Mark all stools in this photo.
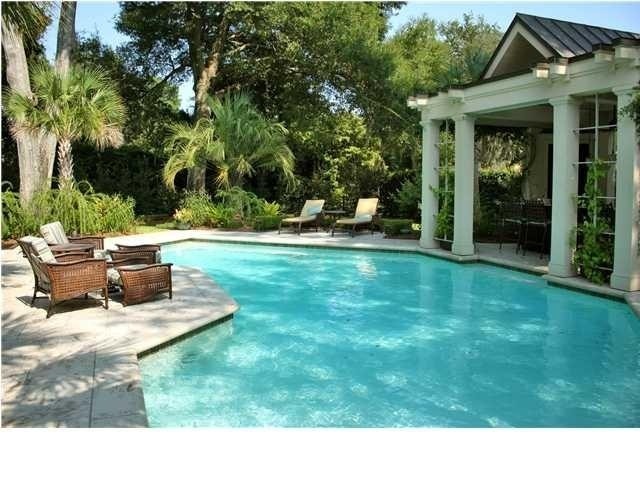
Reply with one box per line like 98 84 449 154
106 243 174 307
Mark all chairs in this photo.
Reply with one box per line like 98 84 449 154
499 198 551 259
278 197 380 238
18 220 108 319
